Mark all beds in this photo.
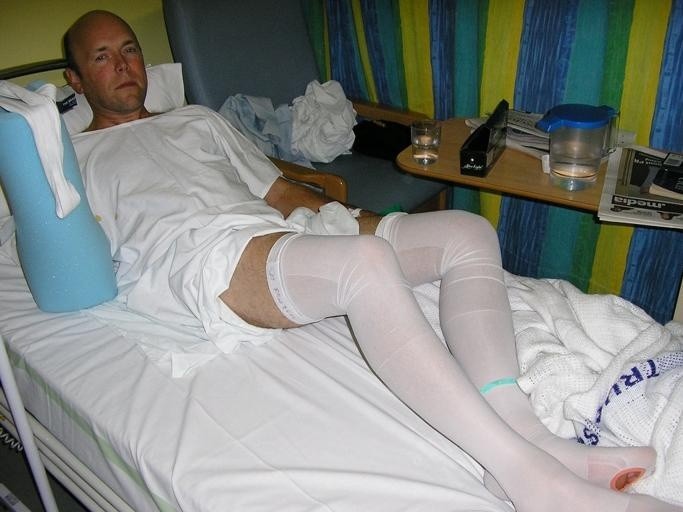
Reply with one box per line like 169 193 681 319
0 59 683 512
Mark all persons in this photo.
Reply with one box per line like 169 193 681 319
611 205 632 212
657 212 680 220
61 8 683 512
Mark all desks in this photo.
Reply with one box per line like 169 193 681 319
397 117 606 210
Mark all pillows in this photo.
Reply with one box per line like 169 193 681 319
55 63 185 133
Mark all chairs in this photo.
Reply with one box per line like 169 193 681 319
163 0 451 217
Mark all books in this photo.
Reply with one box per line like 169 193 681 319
648 152 683 201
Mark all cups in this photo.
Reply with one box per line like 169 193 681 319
410 117 441 166
532 101 619 194
